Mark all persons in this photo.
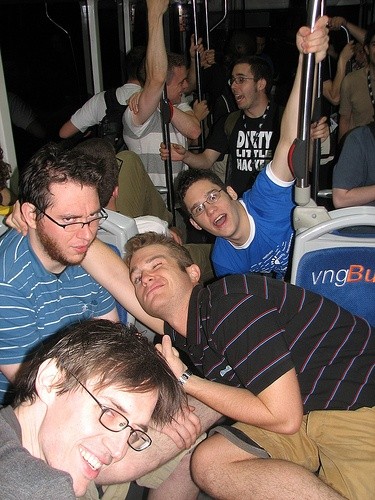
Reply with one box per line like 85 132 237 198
0 142 179 410
4 199 375 500
0 0 375 282
0 319 223 500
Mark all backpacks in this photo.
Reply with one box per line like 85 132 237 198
94 89 128 153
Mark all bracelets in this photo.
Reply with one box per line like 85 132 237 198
175 369 192 386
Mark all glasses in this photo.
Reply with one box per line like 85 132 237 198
189 187 225 218
61 364 152 453
38 208 109 233
227 76 254 86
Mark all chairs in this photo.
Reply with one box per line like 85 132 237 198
290 214 375 327
